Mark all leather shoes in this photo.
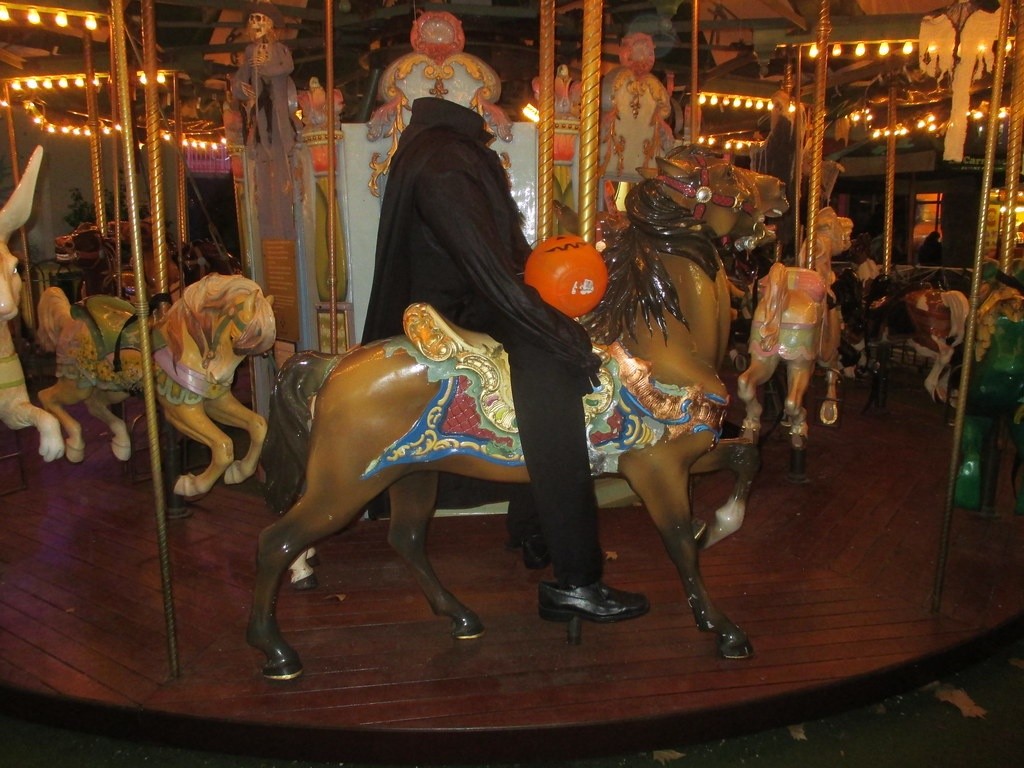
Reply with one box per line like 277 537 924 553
537 581 651 623
523 536 550 570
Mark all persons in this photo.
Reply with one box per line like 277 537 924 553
361 94 650 623
918 230 943 268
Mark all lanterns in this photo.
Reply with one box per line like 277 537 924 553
523 233 609 318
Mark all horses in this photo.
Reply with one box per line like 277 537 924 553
238 146 791 682
724 206 1024 521
33 271 278 497
53 218 235 307
